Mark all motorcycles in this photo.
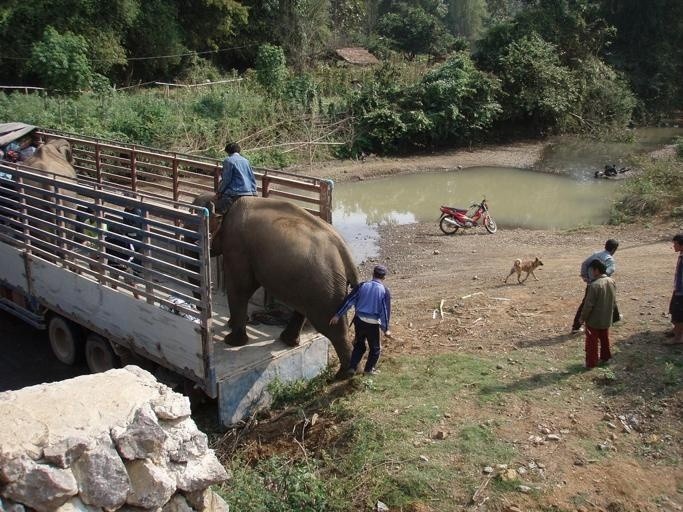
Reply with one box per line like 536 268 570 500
438 194 497 235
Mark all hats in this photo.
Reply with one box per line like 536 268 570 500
374 265 386 274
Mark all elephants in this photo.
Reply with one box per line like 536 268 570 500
8 138 83 276
183 190 365 380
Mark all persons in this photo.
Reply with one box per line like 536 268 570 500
194 142 258 255
577 259 617 369
662 233 682 346
328 264 390 376
80 205 107 288
118 192 143 283
106 224 141 301
570 238 623 334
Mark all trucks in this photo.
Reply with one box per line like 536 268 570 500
1 120 349 435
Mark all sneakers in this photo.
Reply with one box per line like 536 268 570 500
571 328 583 333
347 369 356 376
664 327 678 335
364 368 380 375
664 336 682 345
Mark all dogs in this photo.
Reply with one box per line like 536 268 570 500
504 256 544 284
